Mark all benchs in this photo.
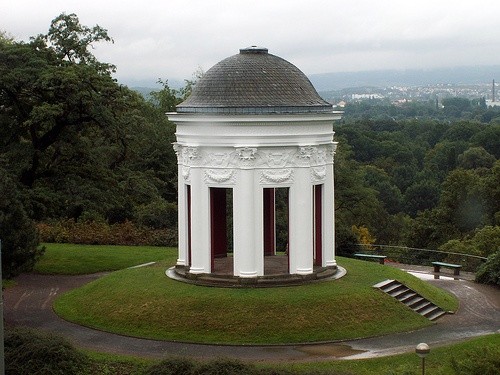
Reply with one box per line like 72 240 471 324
432 260 462 275
354 253 386 264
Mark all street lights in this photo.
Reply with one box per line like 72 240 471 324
416 343 430 375
